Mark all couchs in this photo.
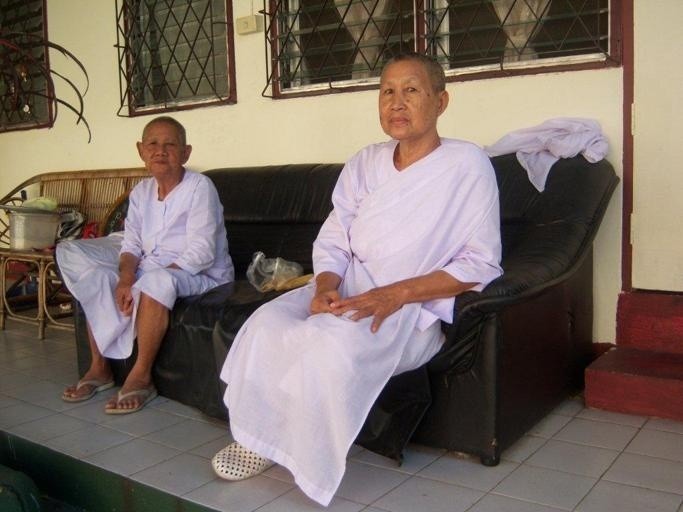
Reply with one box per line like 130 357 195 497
52 147 619 465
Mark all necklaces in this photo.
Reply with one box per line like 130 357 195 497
157 178 181 200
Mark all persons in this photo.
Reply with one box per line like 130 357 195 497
210 50 505 507
53 116 236 414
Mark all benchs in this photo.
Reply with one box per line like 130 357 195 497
0 165 159 342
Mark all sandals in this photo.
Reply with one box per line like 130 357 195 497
212 441 276 482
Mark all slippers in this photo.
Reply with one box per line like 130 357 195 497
104 386 157 414
62 376 114 402
